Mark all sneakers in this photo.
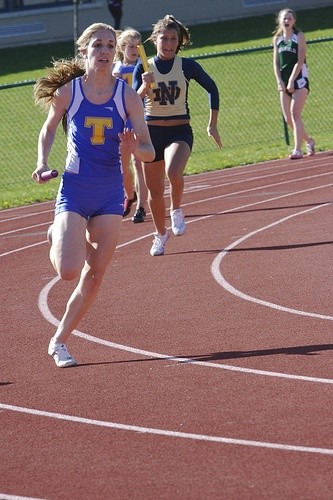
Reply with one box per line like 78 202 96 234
149 226 169 257
123 191 137 217
47 338 77 368
131 206 146 223
289 149 303 159
170 208 186 236
306 138 315 156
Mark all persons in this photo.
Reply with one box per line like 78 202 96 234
111 28 148 223
107 0 122 28
272 9 315 160
30 23 155 368
132 15 222 256
